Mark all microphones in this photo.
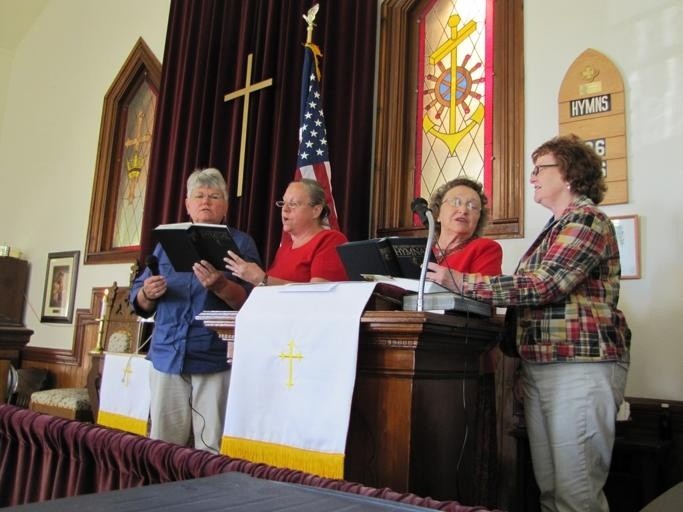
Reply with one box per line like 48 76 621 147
147 255 160 278
411 196 431 230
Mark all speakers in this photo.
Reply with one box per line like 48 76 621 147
604 395 683 511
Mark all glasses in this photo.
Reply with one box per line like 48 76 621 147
274 200 320 209
440 196 481 214
530 164 558 177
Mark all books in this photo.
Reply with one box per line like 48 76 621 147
151 221 245 274
399 290 494 321
335 235 438 282
358 272 455 303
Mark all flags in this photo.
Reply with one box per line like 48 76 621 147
277 40 342 249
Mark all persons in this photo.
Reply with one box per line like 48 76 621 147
127 166 264 458
221 177 350 288
419 173 503 279
419 132 633 512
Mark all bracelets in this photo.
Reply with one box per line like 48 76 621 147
258 272 268 287
141 288 155 302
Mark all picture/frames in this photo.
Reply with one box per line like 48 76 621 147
608 214 641 280
40 251 80 324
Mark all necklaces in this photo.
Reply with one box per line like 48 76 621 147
433 234 476 259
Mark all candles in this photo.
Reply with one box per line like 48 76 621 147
100 289 109 320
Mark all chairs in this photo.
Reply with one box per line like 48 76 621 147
29 330 130 423
16 369 49 409
